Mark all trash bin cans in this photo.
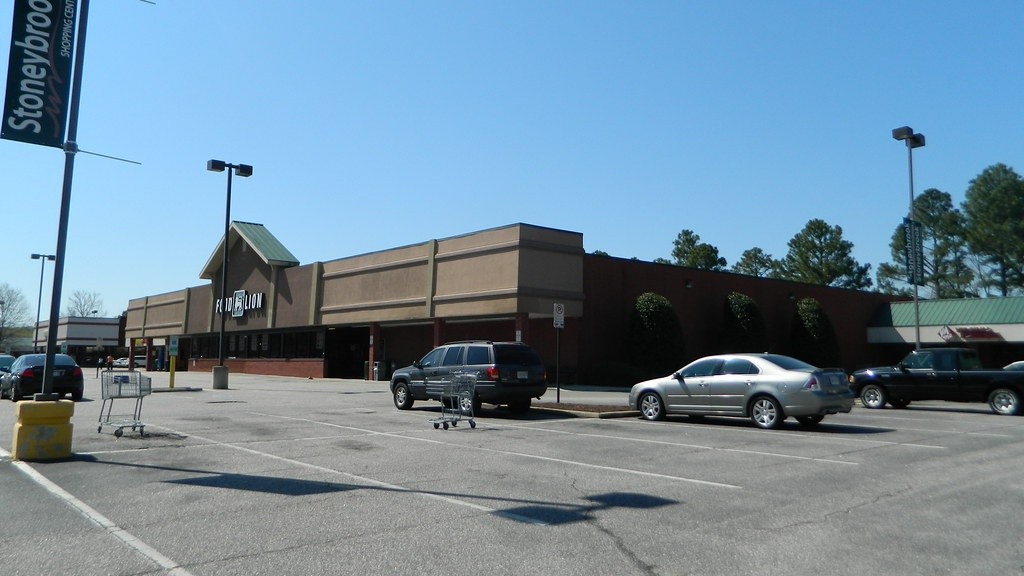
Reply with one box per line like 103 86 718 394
391 362 398 375
364 361 369 380
164 360 170 372
374 361 385 381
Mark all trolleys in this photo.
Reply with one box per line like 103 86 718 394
425 370 477 431
95 370 153 438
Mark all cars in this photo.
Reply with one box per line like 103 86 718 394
0 354 16 378
628 352 854 431
1003 361 1024 371
0 354 85 401
113 357 127 367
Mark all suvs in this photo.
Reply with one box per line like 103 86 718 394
392 340 550 412
851 347 1024 418
127 356 147 368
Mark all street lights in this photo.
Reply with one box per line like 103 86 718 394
890 125 926 350
30 254 57 352
208 157 254 391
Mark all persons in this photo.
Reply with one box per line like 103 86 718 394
107 353 113 370
98 353 105 369
